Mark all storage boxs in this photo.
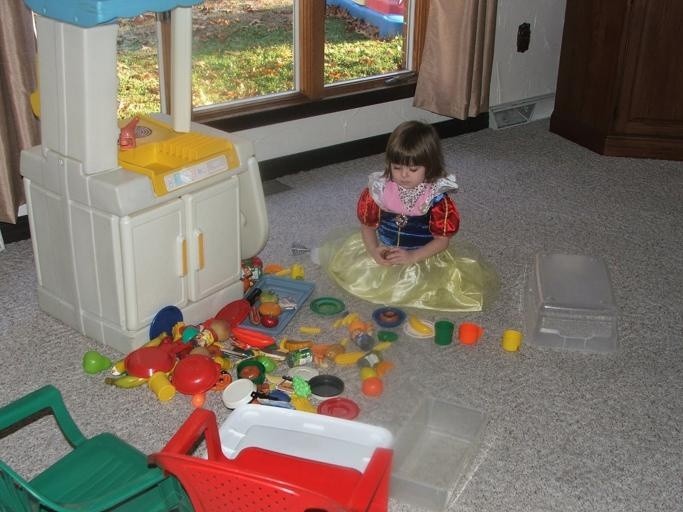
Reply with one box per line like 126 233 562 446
522 252 621 354
384 395 488 512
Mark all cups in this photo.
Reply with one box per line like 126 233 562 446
459 324 478 344
503 330 523 352
435 321 453 345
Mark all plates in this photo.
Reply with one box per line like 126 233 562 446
403 319 436 338
371 307 404 327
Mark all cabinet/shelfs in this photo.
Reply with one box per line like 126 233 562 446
21 116 244 353
548 1 682 162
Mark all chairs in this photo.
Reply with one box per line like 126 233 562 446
149 403 392 511
0 385 194 511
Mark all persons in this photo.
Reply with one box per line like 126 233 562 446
319 119 498 312
